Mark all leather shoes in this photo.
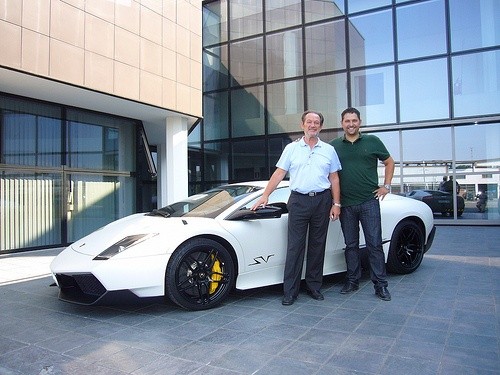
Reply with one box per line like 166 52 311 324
282 294 297 305
308 290 324 299
340 281 359 294
375 287 391 301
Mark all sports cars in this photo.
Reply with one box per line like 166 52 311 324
49 180 437 311
401 189 466 218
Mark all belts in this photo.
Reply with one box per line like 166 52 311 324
291 189 329 197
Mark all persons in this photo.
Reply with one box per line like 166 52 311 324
250 111 343 305
476 190 488 213
294 107 395 302
438 175 462 217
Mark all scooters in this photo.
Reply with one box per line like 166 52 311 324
474 194 487 213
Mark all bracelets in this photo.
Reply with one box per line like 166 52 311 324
383 184 391 189
333 202 341 206
